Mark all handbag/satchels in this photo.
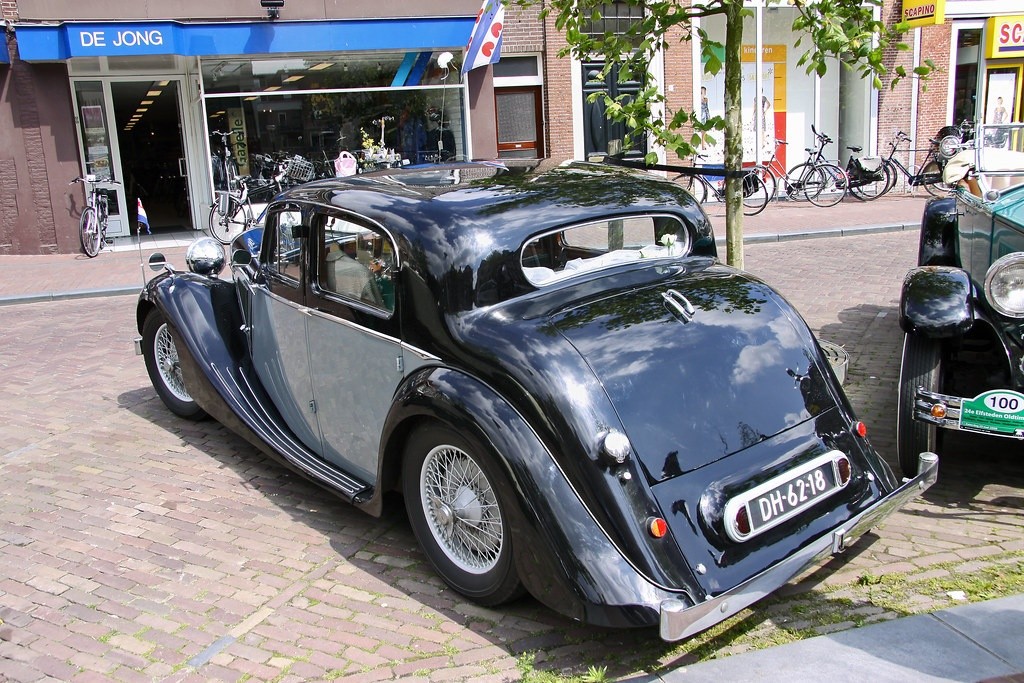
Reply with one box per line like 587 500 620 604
335 150 357 177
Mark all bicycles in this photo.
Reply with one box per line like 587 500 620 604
667 149 768 213
750 139 848 206
802 121 1012 202
67 175 122 258
200 128 352 248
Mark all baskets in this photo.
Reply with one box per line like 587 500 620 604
287 158 314 182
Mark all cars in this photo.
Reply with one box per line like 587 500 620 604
133 153 941 650
899 121 1024 485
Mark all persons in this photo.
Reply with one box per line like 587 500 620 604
991 97 1008 148
701 86 710 150
753 87 771 149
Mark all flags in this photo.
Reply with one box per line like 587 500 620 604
138 198 151 235
